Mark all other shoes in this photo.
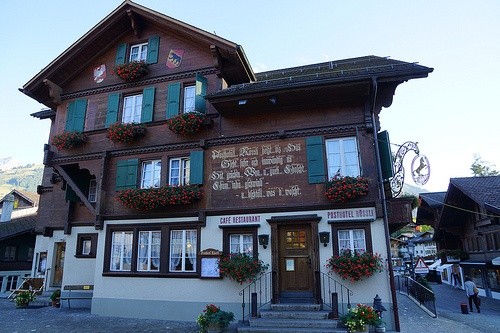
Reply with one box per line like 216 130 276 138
470 310 473 312
478 310 480 313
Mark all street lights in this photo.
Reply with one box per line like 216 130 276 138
406 240 416 281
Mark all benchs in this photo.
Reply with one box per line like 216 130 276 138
56 285 94 310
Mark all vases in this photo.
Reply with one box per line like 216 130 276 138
207 324 222 333
356 325 369 333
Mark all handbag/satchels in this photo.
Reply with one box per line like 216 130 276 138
473 287 479 294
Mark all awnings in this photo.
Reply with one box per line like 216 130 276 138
436 264 453 271
428 259 442 270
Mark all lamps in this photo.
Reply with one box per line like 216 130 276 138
319 232 330 247
259 235 269 249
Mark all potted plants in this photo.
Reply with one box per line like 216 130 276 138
14 290 37 309
50 290 61 308
376 318 385 333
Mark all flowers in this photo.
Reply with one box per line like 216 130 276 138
114 184 201 211
323 248 383 282
338 302 379 333
322 172 369 203
105 121 147 145
169 110 207 138
51 131 89 152
217 253 270 285
196 303 236 333
110 59 149 81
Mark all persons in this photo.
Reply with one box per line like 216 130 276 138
464 276 481 313
